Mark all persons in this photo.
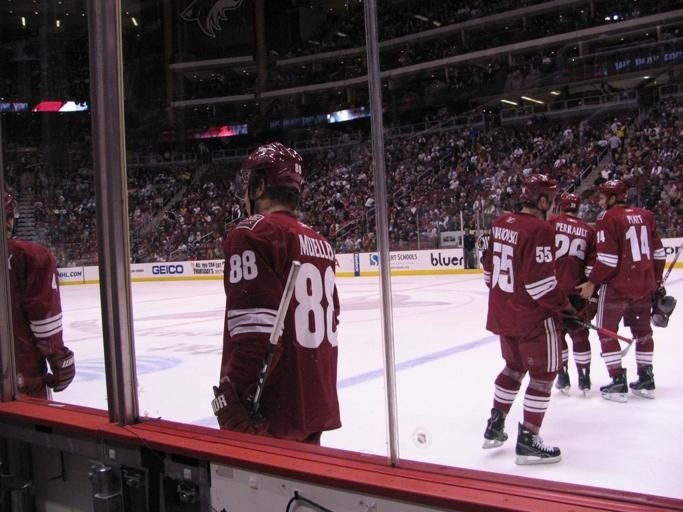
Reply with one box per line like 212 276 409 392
544 190 597 398
608 132 622 159
0 190 76 401
573 178 665 404
210 139 342 446
458 224 477 269
481 174 579 467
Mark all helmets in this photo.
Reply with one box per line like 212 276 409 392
519 173 558 210
0 187 16 234
559 190 581 212
236 140 307 204
597 178 628 203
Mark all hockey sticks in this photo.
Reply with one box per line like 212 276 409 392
248 259 301 419
564 318 633 358
621 248 682 357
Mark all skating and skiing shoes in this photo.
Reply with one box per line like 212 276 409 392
515 426 563 464
599 368 629 403
628 364 656 400
483 409 510 450
577 368 593 399
554 364 572 394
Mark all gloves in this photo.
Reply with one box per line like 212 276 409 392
562 311 579 330
209 375 255 434
42 346 76 393
